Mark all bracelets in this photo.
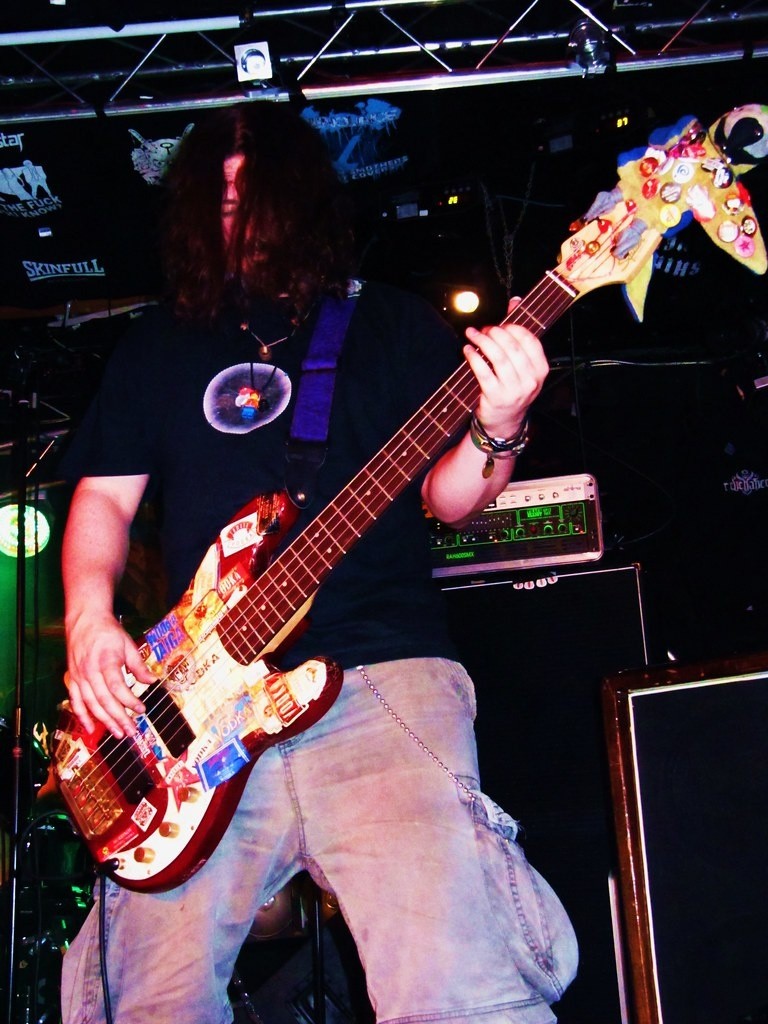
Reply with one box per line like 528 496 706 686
470 416 528 479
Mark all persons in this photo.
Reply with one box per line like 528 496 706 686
47 100 579 1024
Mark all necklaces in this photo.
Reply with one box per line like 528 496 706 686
241 308 312 418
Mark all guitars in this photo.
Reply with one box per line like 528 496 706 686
36 103 768 894
